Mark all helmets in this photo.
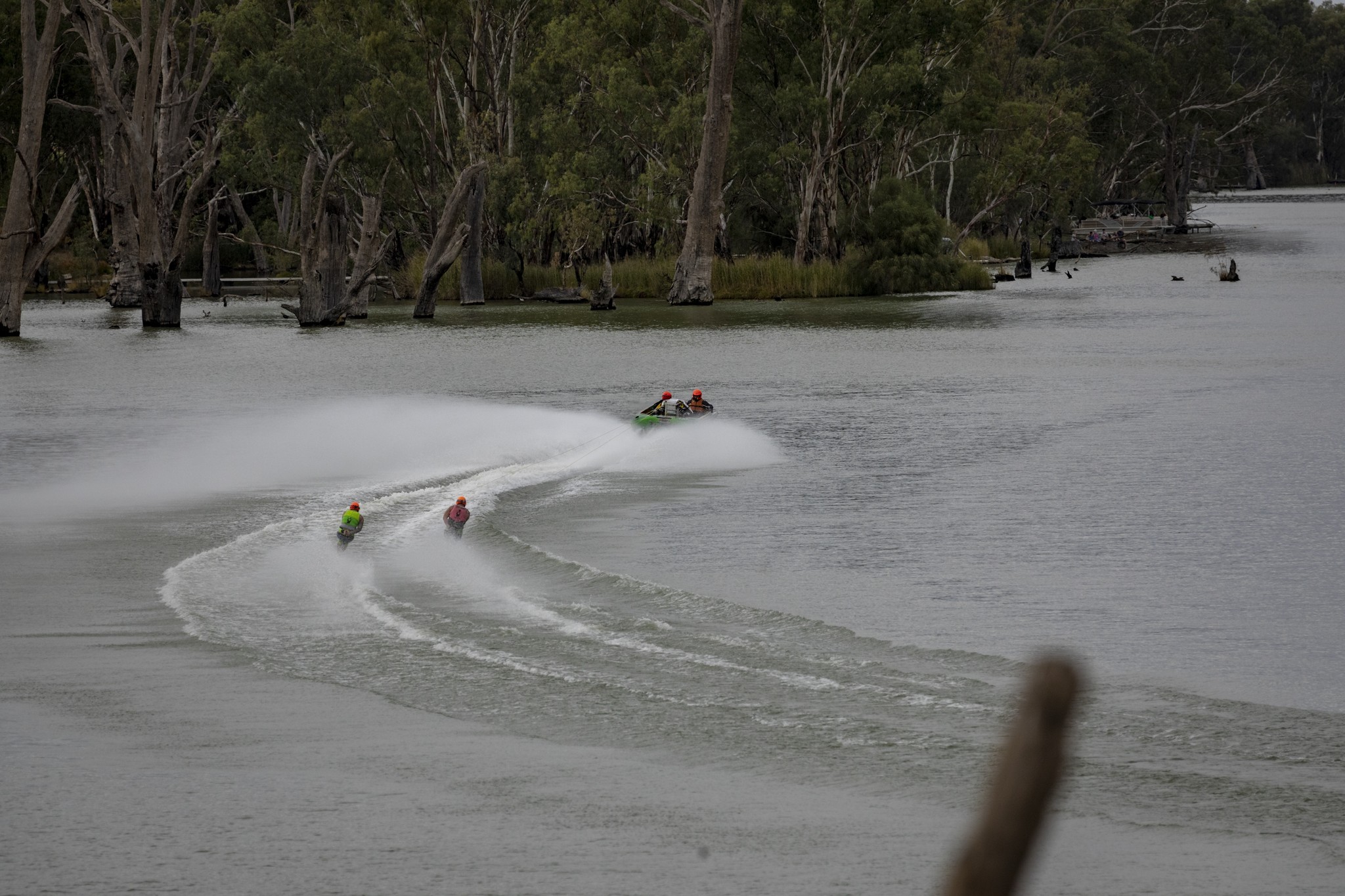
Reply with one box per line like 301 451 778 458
350 502 360 512
662 392 672 400
456 497 466 507
692 389 702 401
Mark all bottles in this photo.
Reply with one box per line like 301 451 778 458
1137 234 1138 239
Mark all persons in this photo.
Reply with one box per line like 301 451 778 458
443 496 470 541
653 392 672 416
683 389 714 418
1135 212 1140 217
1071 229 1079 241
337 502 364 552
1149 213 1154 219
1160 211 1166 219
1087 227 1127 249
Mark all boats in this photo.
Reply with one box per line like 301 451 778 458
630 399 719 430
